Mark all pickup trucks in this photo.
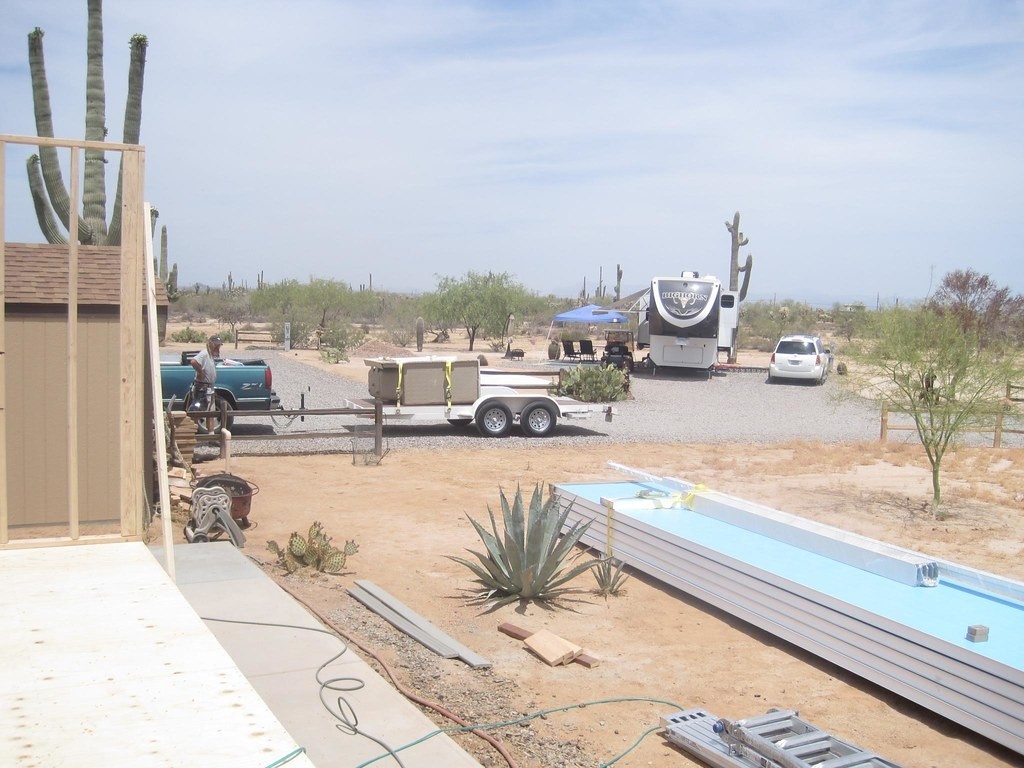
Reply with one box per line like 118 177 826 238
159 358 281 433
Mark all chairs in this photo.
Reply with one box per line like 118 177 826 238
562 340 581 363
579 340 597 363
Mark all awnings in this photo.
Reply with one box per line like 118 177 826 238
592 287 650 312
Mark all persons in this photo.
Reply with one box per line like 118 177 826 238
925 368 936 391
191 335 223 447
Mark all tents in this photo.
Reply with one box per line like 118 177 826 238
540 304 632 360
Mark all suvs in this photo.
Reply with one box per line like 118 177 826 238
765 334 831 387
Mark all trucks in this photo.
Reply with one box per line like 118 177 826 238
639 271 739 379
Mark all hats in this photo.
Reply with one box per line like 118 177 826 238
210 335 224 346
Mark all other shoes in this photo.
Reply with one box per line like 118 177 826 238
209 441 220 446
